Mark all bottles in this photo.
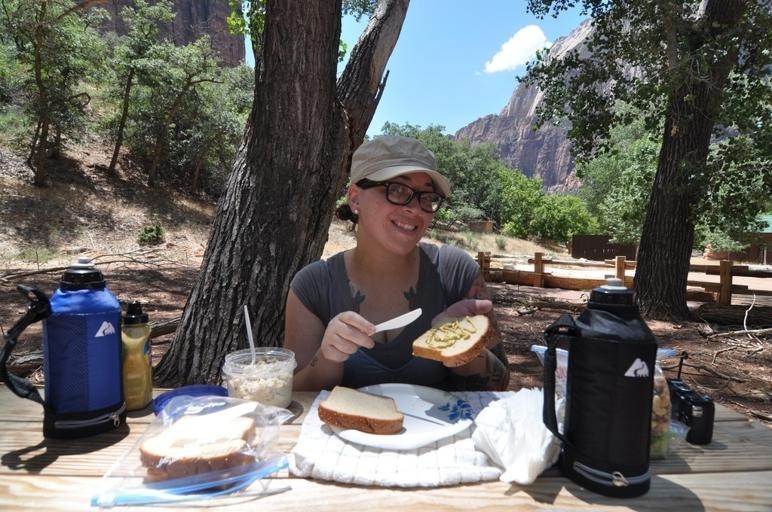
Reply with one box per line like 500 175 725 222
41 256 125 438
121 298 155 410
561 276 661 497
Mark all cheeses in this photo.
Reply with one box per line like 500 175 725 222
228 358 294 409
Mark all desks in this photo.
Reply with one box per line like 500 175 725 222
3 377 770 510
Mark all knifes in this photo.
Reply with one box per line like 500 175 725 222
365 306 424 337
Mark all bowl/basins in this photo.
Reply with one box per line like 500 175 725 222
224 347 305 412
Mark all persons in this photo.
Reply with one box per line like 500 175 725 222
282 133 511 392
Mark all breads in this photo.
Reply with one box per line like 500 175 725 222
317 384 404 434
412 314 492 368
138 399 263 492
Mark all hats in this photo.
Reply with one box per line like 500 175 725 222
349 136 450 200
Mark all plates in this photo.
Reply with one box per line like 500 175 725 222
332 383 473 451
153 384 230 416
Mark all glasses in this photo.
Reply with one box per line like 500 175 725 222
360 181 445 213
